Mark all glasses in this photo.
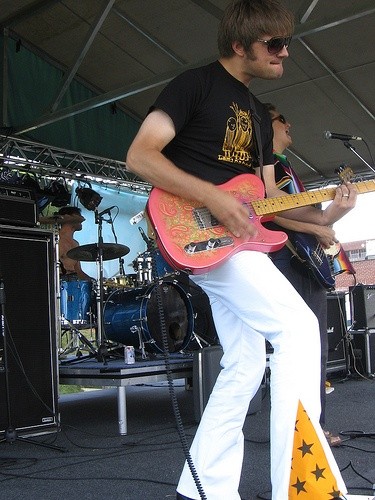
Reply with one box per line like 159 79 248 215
256 35 292 55
272 114 285 124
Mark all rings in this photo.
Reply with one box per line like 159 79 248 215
343 194 349 197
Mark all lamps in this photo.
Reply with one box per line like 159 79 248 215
0 159 72 207
75 179 103 211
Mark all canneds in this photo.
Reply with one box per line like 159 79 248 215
124 346 136 364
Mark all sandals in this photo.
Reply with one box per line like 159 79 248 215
323 429 342 446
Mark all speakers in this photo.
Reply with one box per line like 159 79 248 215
349 285 375 329
348 330 375 377
0 225 60 439
326 292 350 376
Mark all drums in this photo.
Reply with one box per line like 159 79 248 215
57 276 102 332
41 212 86 226
133 245 182 286
102 273 135 293
106 281 198 356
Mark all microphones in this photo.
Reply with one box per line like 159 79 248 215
97 206 115 219
321 130 362 140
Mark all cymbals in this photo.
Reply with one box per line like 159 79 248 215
66 242 131 262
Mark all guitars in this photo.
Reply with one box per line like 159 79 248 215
143 173 375 275
285 164 357 290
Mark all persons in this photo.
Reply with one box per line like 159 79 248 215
54 207 92 279
125 0 357 500
257 101 339 448
